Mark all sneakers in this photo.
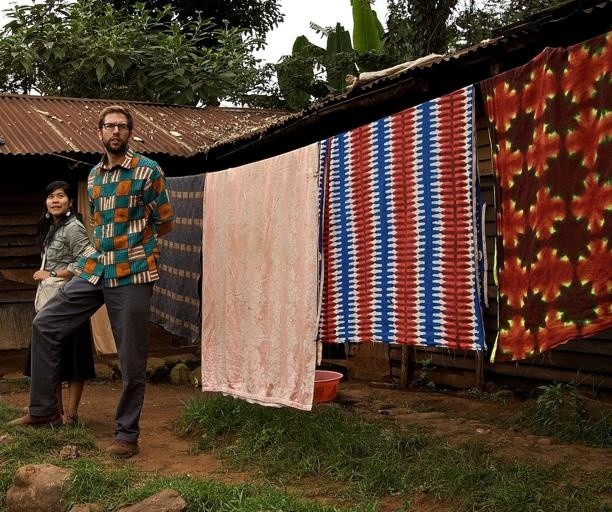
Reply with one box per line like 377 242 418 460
59 413 79 431
107 440 139 458
7 411 64 427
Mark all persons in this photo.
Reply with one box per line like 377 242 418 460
21 181 97 429
6 105 175 459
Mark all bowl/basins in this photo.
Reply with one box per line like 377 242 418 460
300 368 344 403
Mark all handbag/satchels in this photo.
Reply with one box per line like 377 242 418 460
34 276 68 313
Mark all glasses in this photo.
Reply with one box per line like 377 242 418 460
103 122 128 132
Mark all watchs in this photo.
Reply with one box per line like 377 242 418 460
50 270 57 278
